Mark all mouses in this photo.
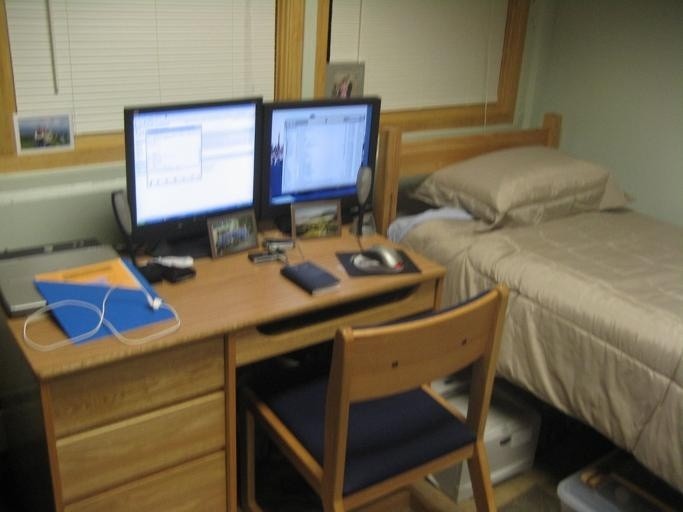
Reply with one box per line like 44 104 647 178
360 240 406 274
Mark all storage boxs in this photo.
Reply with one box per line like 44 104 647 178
427 383 543 503
557 453 681 512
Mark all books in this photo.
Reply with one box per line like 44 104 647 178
280 260 342 298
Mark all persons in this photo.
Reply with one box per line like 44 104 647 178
33 126 53 147
338 72 353 98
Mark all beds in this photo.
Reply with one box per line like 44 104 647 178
375 111 683 493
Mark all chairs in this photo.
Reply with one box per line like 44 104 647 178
239 283 509 512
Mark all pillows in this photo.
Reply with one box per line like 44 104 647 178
411 146 638 233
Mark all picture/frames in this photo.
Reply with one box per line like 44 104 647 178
13 110 75 157
206 208 259 259
324 63 365 100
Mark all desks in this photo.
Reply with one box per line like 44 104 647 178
0 224 447 511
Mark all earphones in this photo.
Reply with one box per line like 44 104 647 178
147 292 163 310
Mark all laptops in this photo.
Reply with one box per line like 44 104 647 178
1 242 122 320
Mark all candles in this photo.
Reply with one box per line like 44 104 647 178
290 199 342 240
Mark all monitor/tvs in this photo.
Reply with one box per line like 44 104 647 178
263 95 382 234
123 98 263 259
